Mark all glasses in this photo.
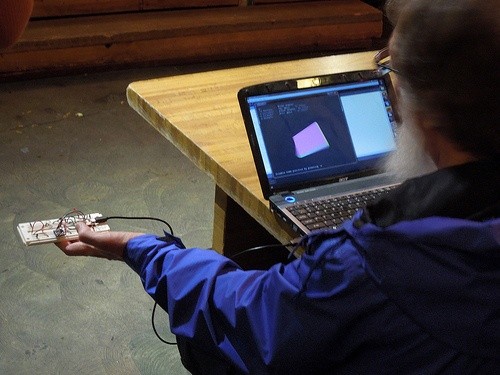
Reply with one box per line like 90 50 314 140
372 44 413 81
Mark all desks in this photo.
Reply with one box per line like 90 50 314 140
126 51 396 260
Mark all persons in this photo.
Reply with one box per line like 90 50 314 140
52 0 500 375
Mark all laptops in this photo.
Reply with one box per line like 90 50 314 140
237 69 405 237
